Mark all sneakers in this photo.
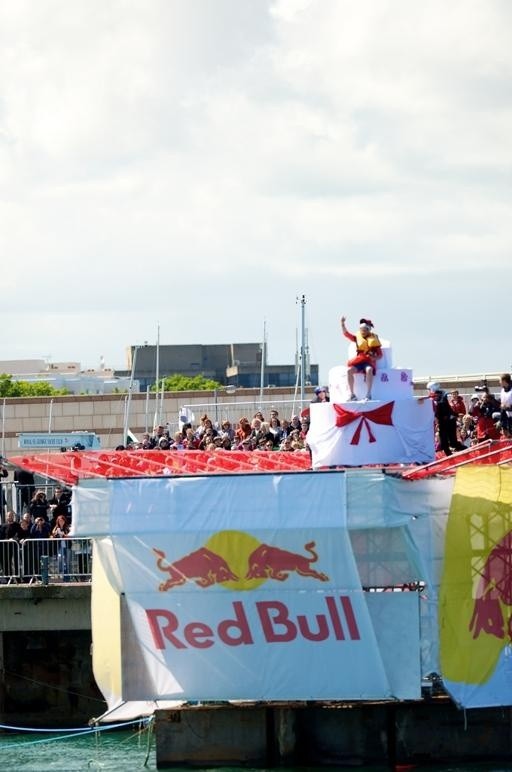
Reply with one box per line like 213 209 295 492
346 393 372 401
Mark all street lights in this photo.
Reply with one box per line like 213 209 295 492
215 385 236 422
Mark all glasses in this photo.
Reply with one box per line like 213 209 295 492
160 414 308 447
319 390 325 392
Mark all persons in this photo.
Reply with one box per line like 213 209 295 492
1 463 91 583
425 373 512 457
340 316 383 402
115 385 330 452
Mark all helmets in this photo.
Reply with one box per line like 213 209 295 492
426 381 440 393
314 386 326 393
359 322 372 331
